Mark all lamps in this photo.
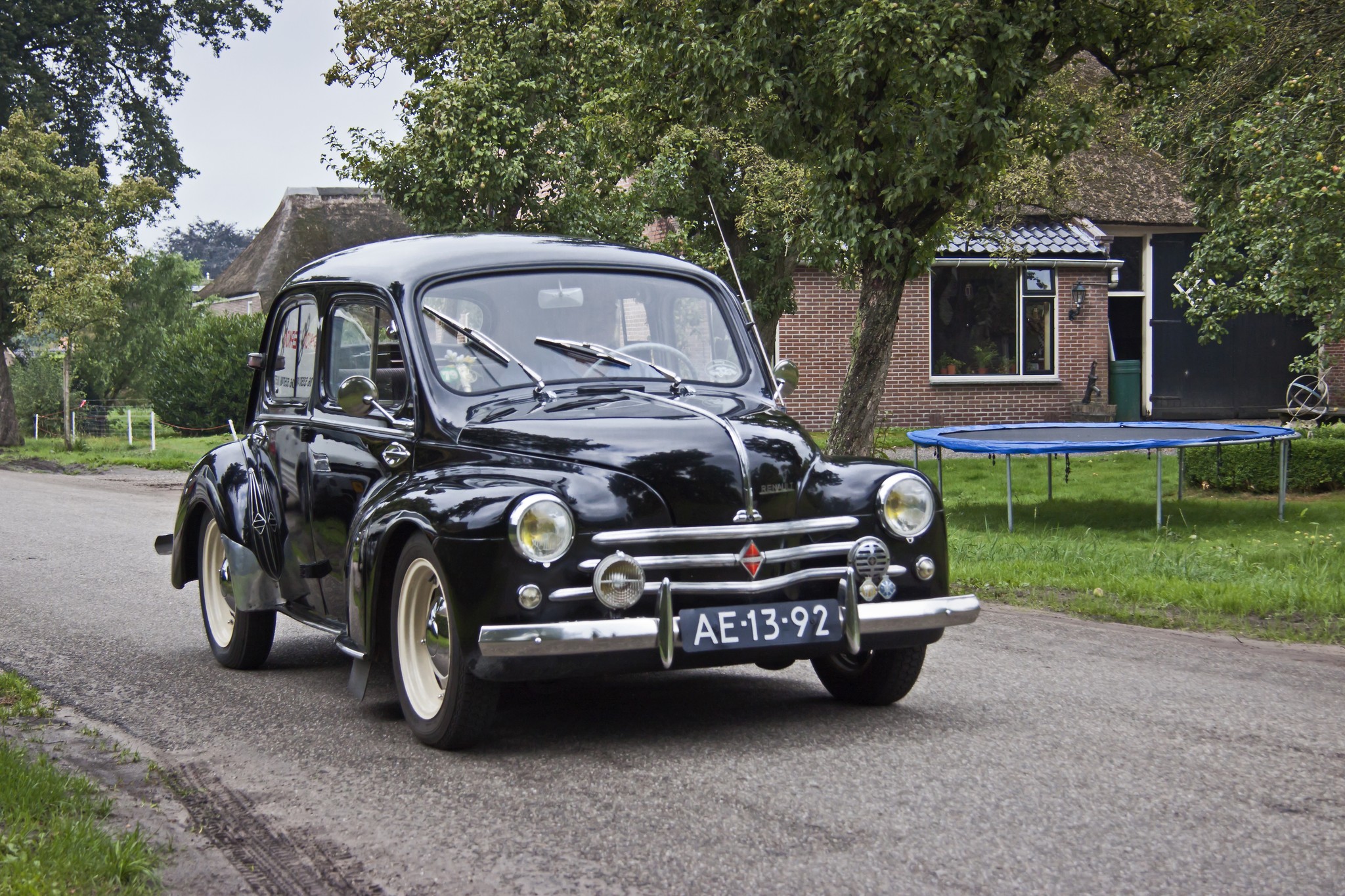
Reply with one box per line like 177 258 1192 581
1069 277 1087 321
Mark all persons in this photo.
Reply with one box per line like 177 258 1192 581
541 277 673 379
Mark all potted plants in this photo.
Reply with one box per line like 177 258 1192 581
930 340 1036 376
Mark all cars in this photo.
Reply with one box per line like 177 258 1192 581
155 195 979 752
